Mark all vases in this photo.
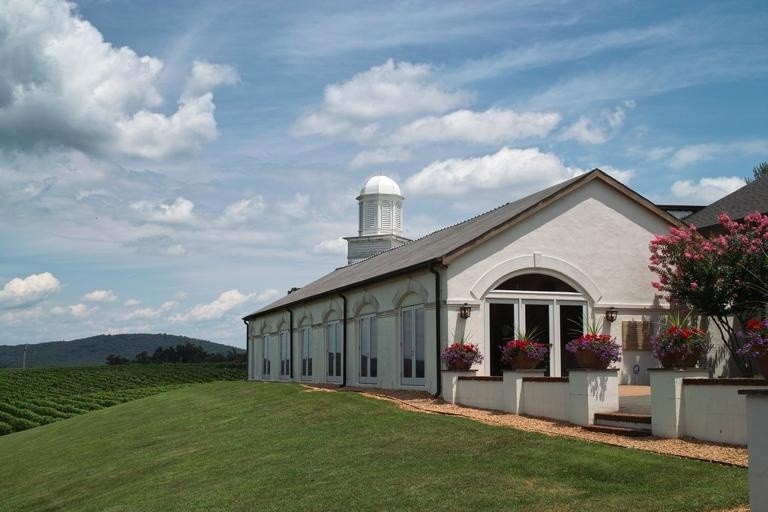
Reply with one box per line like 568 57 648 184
443 359 475 373
571 344 615 372
664 344 703 371
752 346 768 382
506 349 540 371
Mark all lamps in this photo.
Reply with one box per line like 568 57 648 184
459 301 473 320
604 305 619 323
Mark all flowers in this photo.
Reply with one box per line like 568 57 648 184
439 338 486 364
653 319 709 359
563 331 621 361
498 333 550 364
736 311 768 359
